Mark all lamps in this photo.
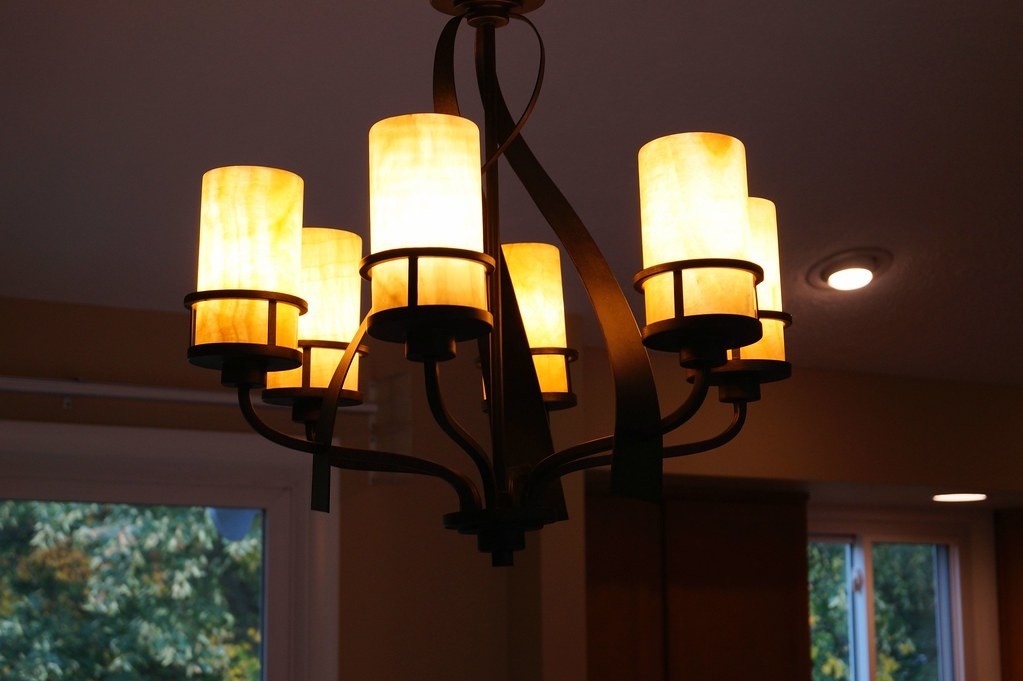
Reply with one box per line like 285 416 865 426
804 243 906 292
184 0 791 570
